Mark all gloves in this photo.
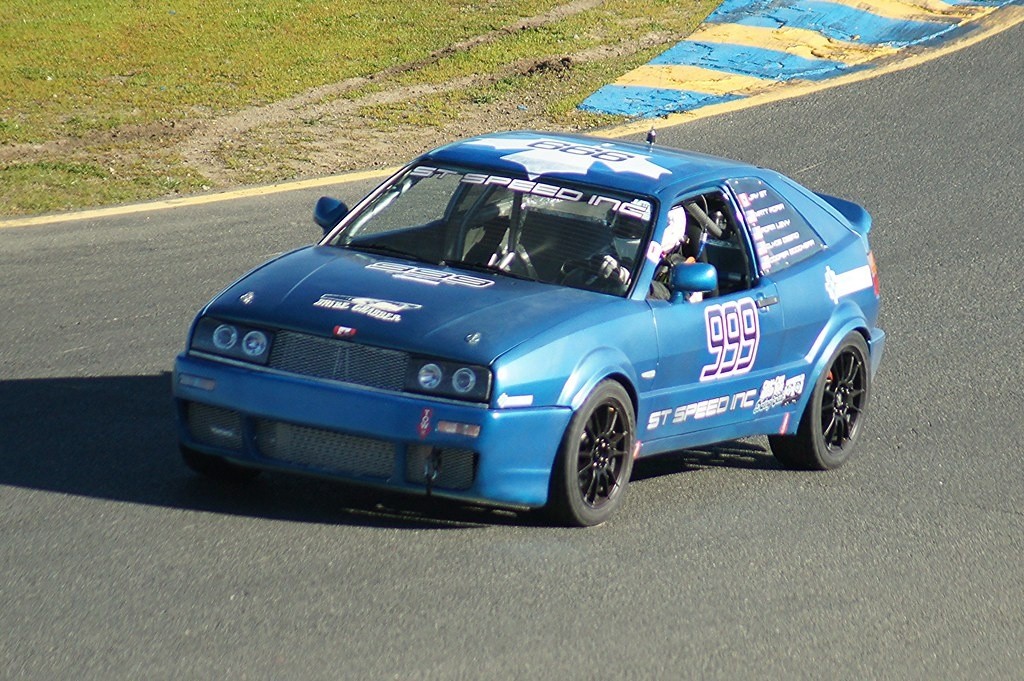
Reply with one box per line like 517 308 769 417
589 254 632 287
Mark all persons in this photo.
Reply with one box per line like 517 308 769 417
581 199 697 306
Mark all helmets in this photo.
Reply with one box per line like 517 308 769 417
606 199 687 265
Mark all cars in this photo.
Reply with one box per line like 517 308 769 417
169 127 888 529
